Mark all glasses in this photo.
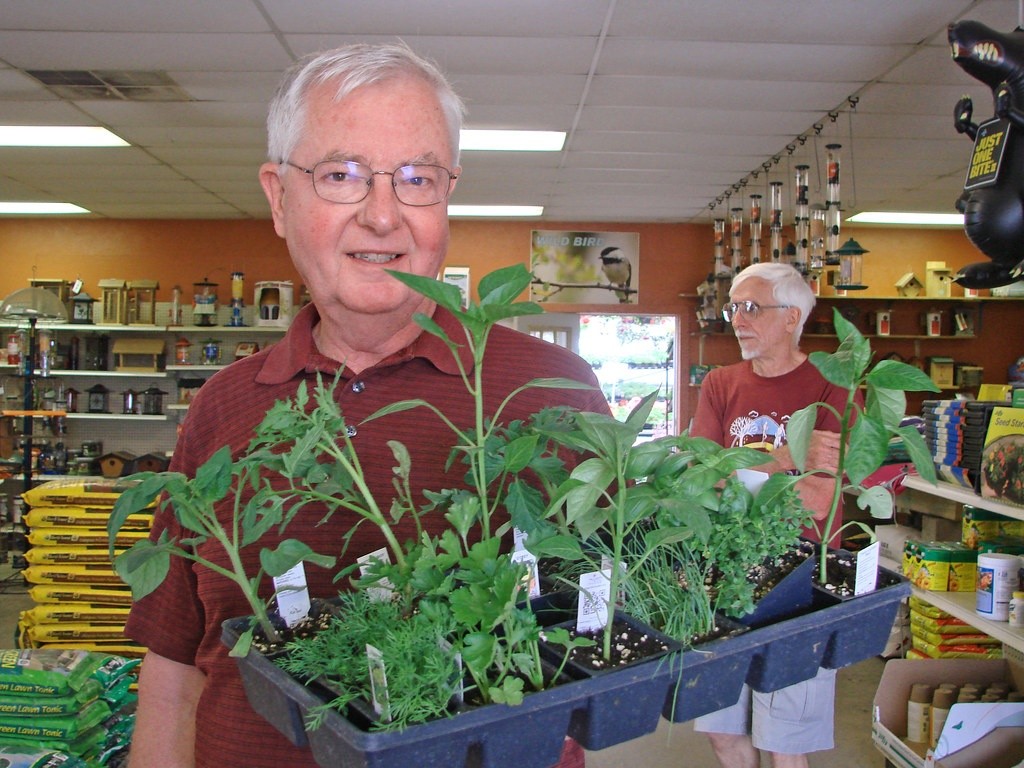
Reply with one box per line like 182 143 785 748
278 155 458 208
721 300 791 323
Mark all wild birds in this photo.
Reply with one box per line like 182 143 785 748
598 246 632 302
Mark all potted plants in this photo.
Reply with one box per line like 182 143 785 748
108 265 945 768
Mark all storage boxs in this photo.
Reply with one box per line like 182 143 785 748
872 657 1024 768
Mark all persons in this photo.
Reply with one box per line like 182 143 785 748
125 41 635 768
690 262 864 768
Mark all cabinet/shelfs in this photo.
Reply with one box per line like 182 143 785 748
677 292 1024 665
0 319 288 572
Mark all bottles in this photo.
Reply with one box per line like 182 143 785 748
1009 592 1024 626
8 335 18 364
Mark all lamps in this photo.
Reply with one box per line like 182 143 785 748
0 284 70 593
715 143 870 291
0 276 299 536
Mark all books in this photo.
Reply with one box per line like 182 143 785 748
923 400 1013 490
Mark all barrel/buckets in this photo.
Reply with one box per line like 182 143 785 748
975 553 1024 621
